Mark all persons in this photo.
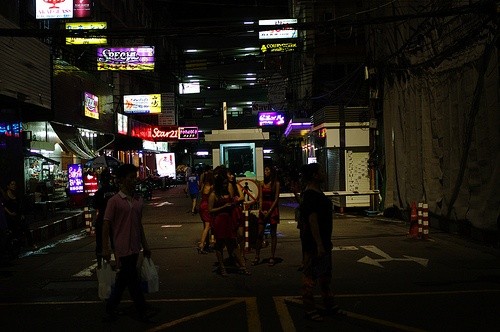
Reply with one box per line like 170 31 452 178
101 163 160 319
0 179 25 259
293 176 308 271
250 163 281 267
293 163 349 324
90 170 117 273
183 163 253 277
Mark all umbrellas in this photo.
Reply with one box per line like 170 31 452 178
80 154 123 170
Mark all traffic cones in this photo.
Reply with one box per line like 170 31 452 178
409 199 419 237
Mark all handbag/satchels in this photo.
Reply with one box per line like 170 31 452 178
143 257 158 291
97 259 112 299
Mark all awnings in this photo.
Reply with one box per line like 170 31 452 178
49 121 97 160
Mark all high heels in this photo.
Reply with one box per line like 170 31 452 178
197 248 206 254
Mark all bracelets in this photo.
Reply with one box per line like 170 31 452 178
224 204 227 208
270 209 272 211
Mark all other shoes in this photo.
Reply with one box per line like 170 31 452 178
252 257 260 263
218 268 226 275
240 267 247 273
